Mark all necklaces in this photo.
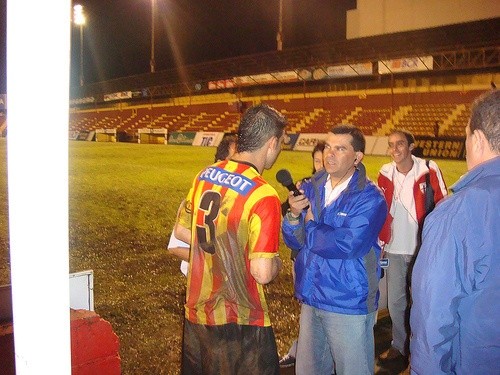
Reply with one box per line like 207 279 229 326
327 172 346 189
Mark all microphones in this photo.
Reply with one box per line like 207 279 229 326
276 169 310 211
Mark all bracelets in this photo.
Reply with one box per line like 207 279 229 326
286 208 299 223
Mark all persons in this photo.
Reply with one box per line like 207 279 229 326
277 143 324 367
282 125 388 375
167 134 239 277
174 104 287 375
376 132 449 362
409 90 500 375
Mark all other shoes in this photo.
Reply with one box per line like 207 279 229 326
279 354 296 367
377 347 409 368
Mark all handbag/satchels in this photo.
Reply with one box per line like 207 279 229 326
424 158 435 213
